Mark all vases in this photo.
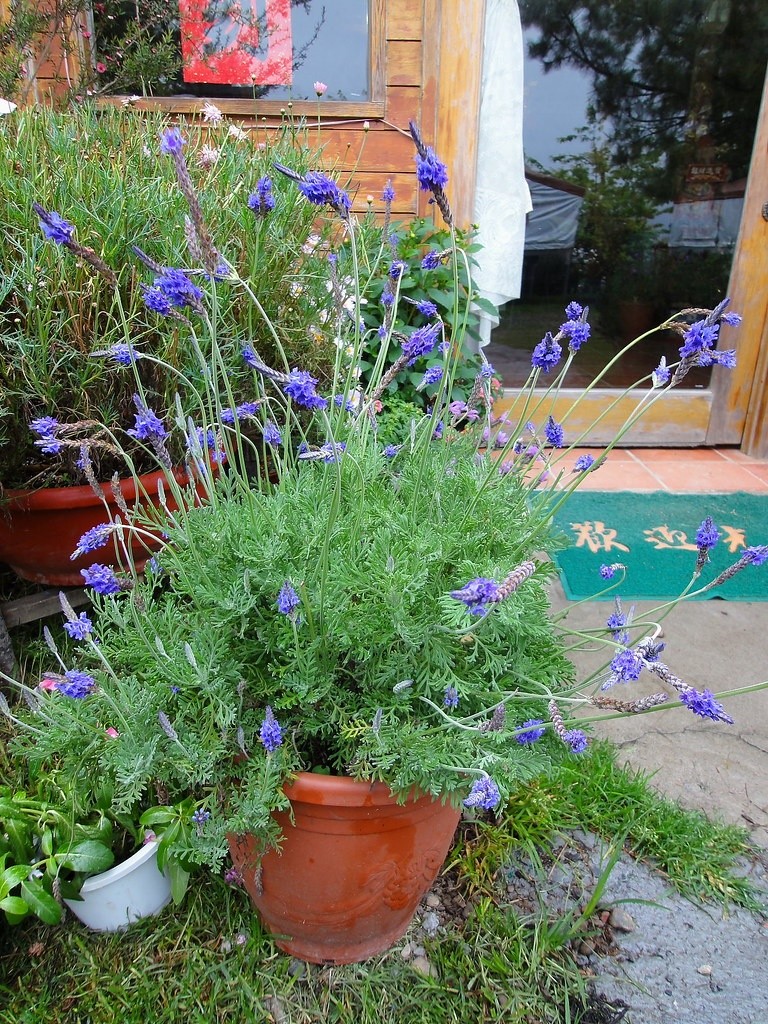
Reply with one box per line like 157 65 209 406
0 424 253 587
213 751 472 965
52 821 190 937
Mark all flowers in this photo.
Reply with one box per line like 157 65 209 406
25 104 764 809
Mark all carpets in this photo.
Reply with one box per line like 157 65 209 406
521 490 768 602
